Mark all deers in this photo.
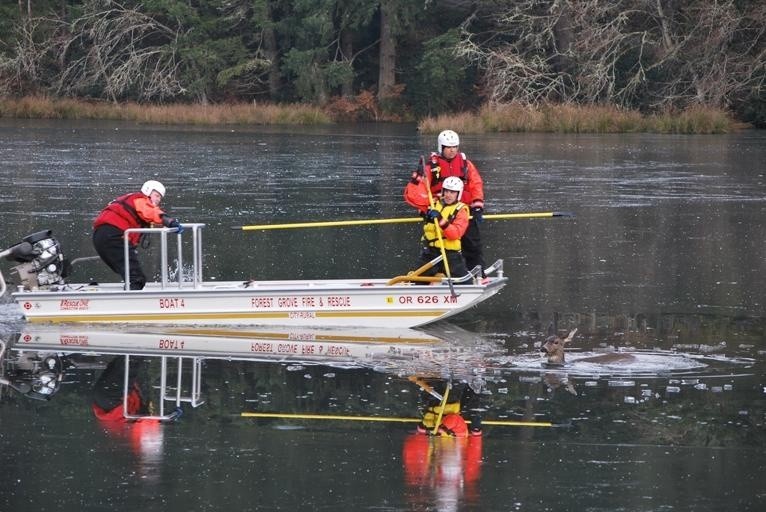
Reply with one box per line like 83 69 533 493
541 322 639 367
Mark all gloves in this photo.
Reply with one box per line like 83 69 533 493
410 165 424 185
159 214 184 234
471 207 484 224
425 209 443 224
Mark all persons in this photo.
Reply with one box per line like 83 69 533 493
92 181 185 291
400 374 484 512
415 173 475 286
405 129 487 280
91 350 184 483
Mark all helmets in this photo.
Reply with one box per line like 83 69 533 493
441 176 464 201
438 129 460 154
140 180 166 199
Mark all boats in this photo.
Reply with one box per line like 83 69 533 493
11 323 500 368
0 223 507 325
